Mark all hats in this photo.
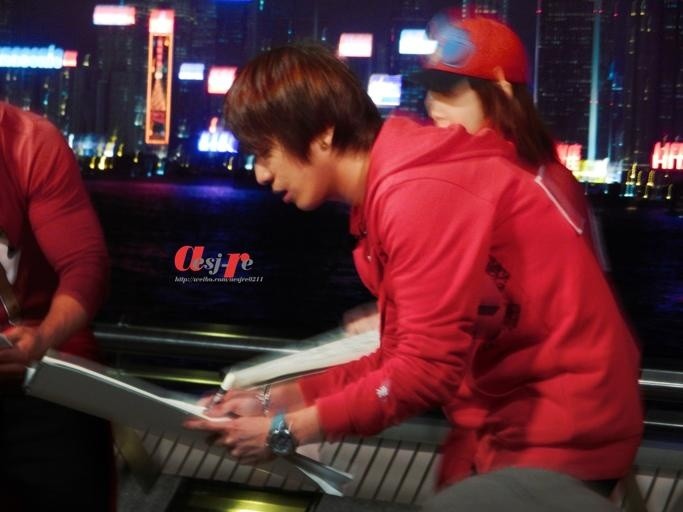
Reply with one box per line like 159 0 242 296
421 15 531 96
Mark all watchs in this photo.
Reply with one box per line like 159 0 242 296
267 412 295 459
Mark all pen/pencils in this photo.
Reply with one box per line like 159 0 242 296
209 371 235 409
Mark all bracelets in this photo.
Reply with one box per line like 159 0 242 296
260 383 270 418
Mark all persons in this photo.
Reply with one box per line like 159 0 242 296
181 46 647 496
342 18 604 336
1 78 111 512
